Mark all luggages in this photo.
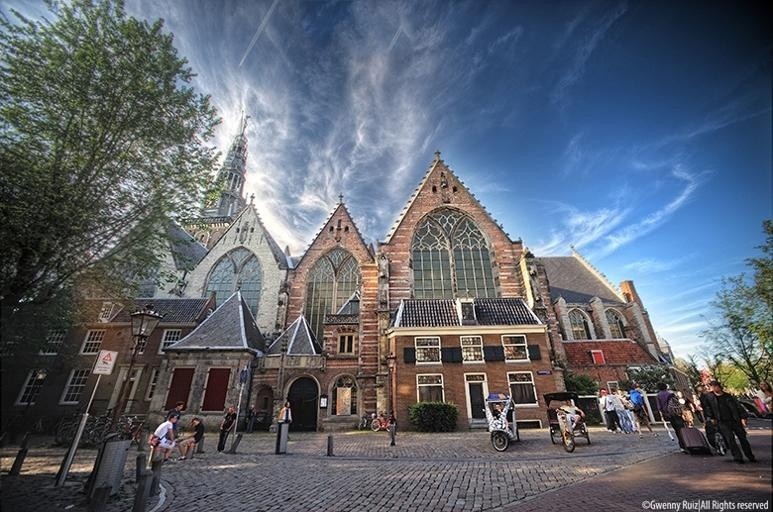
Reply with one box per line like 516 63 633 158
678 421 712 456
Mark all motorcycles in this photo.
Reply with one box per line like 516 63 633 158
358 409 397 433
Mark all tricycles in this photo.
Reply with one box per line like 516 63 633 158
481 393 519 452
541 391 591 453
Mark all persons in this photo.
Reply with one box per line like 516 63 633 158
279 401 293 441
555 399 585 435
148 400 185 462
360 408 397 434
493 403 505 429
596 370 773 464
178 418 204 460
215 404 236 455
246 404 257 435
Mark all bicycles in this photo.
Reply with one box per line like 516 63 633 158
0 407 142 450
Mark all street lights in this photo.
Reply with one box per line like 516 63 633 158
267 286 290 432
107 301 170 437
22 366 49 413
384 349 398 448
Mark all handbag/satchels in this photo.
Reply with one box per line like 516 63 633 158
149 435 161 447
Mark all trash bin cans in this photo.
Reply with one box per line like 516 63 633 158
276 420 289 457
88 432 131 503
